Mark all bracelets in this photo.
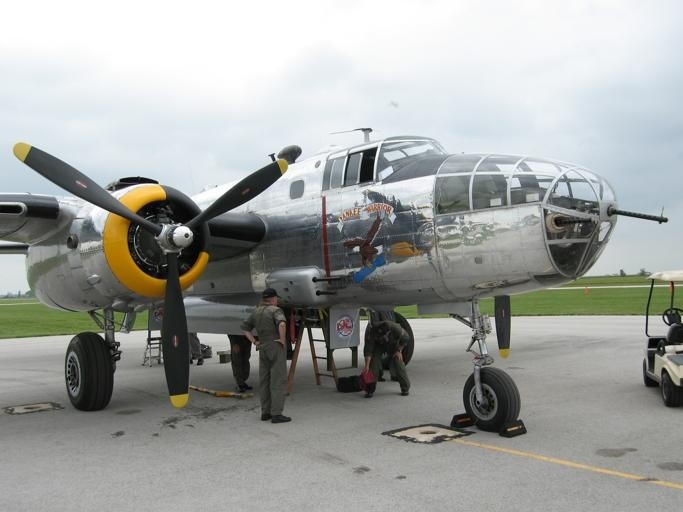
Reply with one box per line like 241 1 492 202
254 339 257 345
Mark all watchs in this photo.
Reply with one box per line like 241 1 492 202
396 350 401 352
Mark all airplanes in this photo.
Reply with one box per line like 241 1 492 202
1 126 670 434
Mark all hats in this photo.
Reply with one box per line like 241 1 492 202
261 288 281 299
373 321 391 337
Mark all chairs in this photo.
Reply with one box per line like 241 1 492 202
666 322 683 345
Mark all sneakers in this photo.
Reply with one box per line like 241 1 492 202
260 413 290 422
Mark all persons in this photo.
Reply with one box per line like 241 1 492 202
227 335 253 392
487 180 497 194
362 320 411 399
190 332 204 366
368 308 397 382
240 288 292 424
